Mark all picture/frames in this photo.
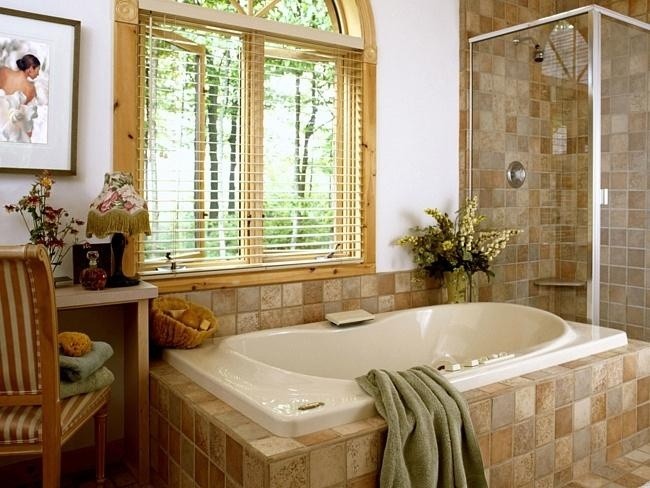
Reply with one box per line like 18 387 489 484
0 6 82 177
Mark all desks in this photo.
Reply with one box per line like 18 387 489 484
51 275 160 487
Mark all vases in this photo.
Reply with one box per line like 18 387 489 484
437 270 472 305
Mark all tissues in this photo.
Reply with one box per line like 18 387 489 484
72 234 112 283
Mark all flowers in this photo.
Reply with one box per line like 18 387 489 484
391 196 522 293
4 172 80 272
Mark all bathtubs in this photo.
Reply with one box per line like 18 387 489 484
163 301 629 436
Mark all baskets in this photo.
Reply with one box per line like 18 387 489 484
152 295 218 347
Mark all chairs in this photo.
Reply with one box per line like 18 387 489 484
1 242 114 488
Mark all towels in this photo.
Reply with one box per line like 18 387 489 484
57 341 114 399
356 365 491 488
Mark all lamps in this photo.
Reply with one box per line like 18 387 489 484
85 169 154 290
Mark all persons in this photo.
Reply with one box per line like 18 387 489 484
0 53 41 142
